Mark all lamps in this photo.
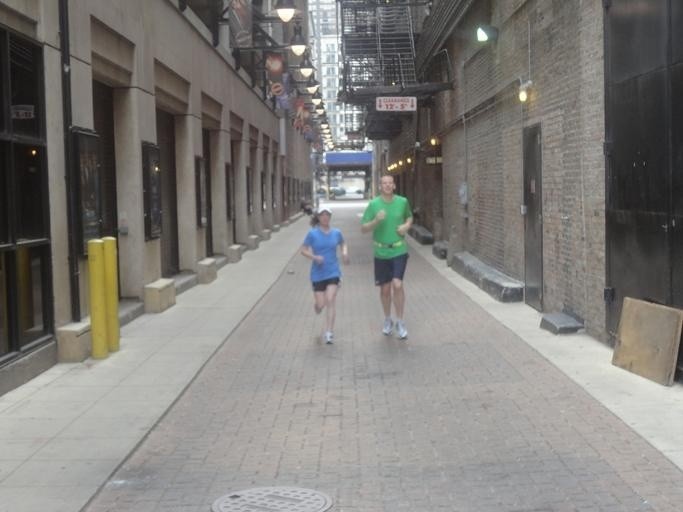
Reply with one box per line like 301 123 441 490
224 0 336 153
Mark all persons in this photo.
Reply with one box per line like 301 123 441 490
358 172 414 340
297 202 349 347
300 198 312 216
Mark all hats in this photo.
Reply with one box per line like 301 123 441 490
317 205 331 215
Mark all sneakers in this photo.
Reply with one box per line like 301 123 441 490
396 321 407 338
383 318 394 335
324 331 334 343
